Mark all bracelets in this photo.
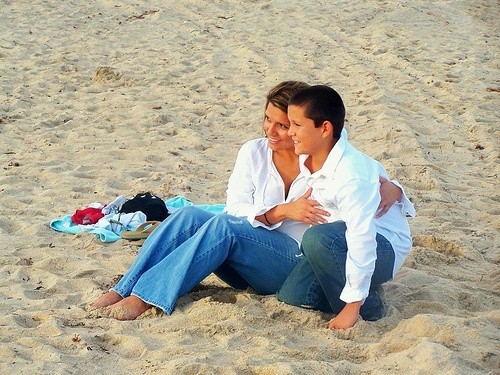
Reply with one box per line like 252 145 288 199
264 213 272 225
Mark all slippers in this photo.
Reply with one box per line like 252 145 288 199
131 239 145 246
121 221 162 239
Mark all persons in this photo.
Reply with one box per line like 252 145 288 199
90 81 417 320
278 86 412 330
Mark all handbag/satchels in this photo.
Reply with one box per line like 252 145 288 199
118 192 171 222
